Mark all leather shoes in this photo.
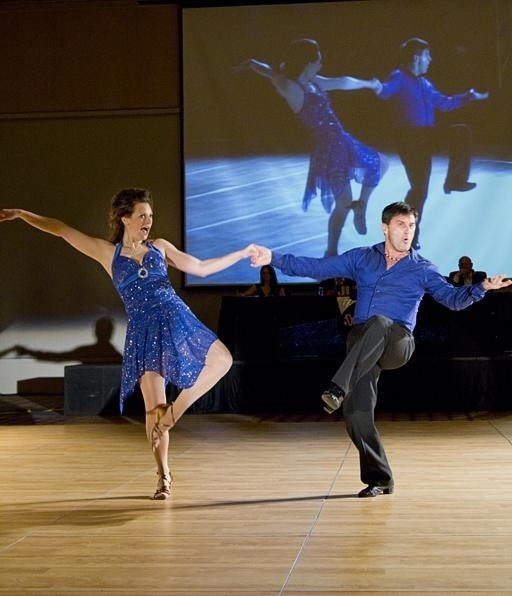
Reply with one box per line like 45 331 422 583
444 182 476 194
321 386 344 414
358 478 394 497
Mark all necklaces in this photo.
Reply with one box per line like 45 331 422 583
384 253 399 262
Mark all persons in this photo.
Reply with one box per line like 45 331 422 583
448 255 486 287
0 187 259 500
251 201 512 498
317 278 357 295
237 265 286 297
238 38 382 258
381 38 489 251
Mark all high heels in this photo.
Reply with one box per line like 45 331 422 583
147 403 175 452
154 472 173 499
345 200 367 234
